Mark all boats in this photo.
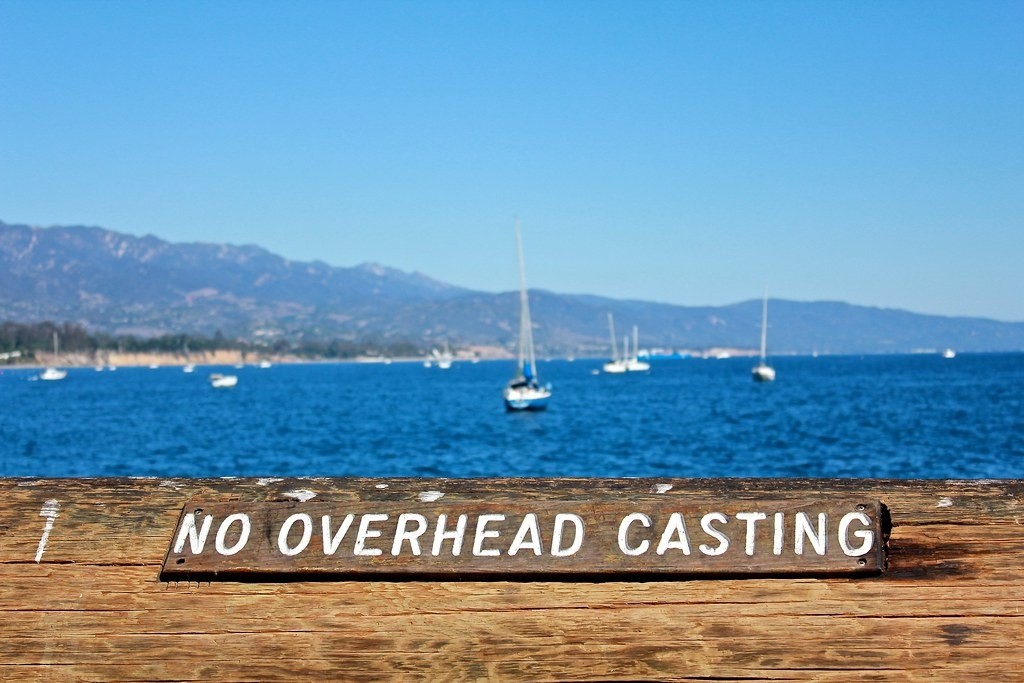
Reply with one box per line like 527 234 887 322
211 376 238 389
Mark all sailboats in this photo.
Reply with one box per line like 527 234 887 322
41 332 67 381
500 212 552 411
750 286 776 383
602 316 648 374
